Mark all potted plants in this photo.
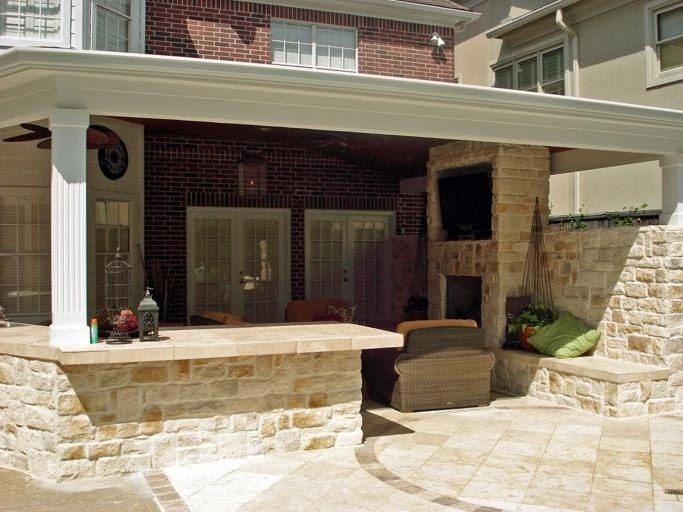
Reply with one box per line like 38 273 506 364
505 302 559 354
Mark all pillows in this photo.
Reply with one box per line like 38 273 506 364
526 310 600 358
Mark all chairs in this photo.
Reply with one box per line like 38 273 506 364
284 298 496 414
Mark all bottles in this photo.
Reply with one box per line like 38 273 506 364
90 318 98 343
